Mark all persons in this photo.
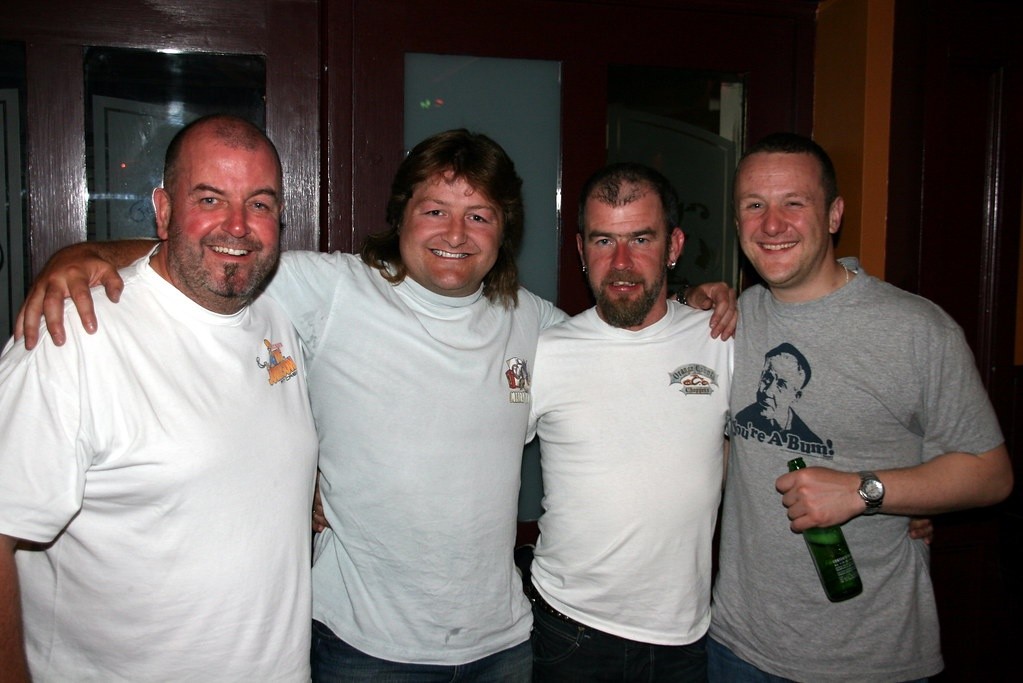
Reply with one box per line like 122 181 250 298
12 131 739 682
703 130 1012 681
0 114 313 681
309 161 937 682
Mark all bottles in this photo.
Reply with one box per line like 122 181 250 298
788 457 864 603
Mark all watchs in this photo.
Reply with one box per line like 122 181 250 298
857 469 885 516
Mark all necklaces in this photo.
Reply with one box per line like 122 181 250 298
840 260 849 285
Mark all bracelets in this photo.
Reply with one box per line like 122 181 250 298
676 282 698 306
